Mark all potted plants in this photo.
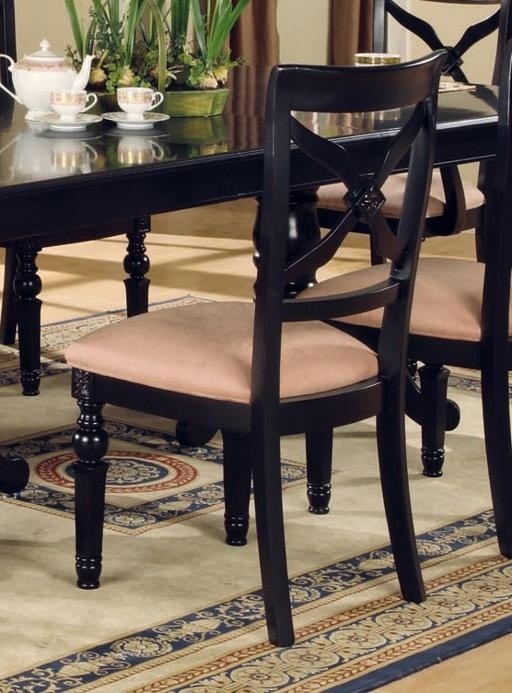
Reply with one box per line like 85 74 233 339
62 2 253 121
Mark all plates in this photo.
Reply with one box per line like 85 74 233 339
101 111 170 130
39 113 103 133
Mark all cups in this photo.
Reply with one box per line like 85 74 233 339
52 141 98 168
47 89 97 123
355 51 402 66
117 137 166 168
116 86 164 120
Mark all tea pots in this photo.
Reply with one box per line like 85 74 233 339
0 40 97 123
3 131 92 177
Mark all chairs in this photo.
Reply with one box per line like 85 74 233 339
312 1 511 265
292 107 512 564
60 44 450 649
2 0 20 357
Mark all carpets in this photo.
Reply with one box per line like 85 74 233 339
2 287 512 690
